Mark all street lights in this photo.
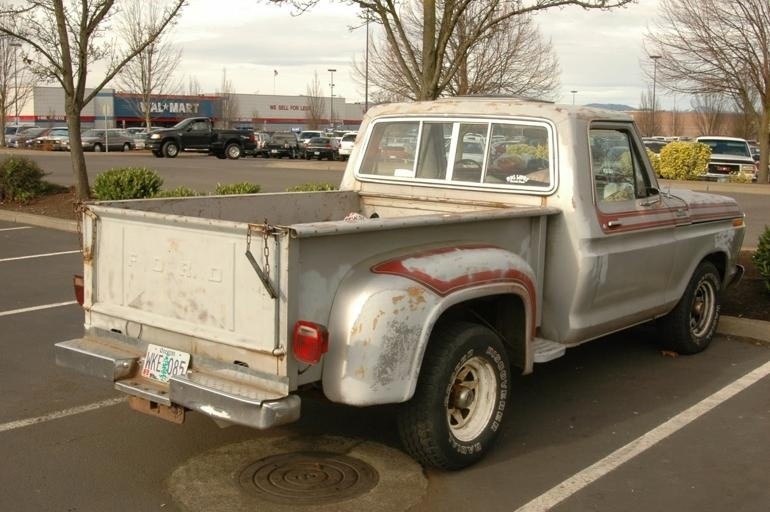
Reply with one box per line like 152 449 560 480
570 91 578 105
644 53 663 130
326 69 337 122
8 44 22 125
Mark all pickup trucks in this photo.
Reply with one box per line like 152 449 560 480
54 95 749 474
